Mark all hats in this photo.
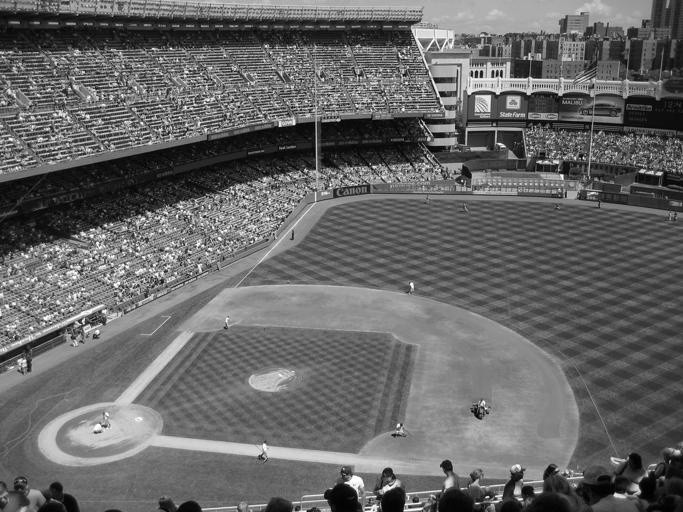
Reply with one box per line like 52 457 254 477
341 466 354 475
440 460 452 467
579 465 611 486
510 464 525 474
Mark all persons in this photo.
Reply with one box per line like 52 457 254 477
1 1 445 235
395 422 408 437
25 352 33 373
407 281 416 296
257 440 271 461
291 229 294 239
472 398 492 414
92 421 102 433
475 406 485 418
99 412 111 428
462 202 466 213
15 356 23 373
20 356 27 376
0 443 682 512
0 236 270 349
522 122 681 220
